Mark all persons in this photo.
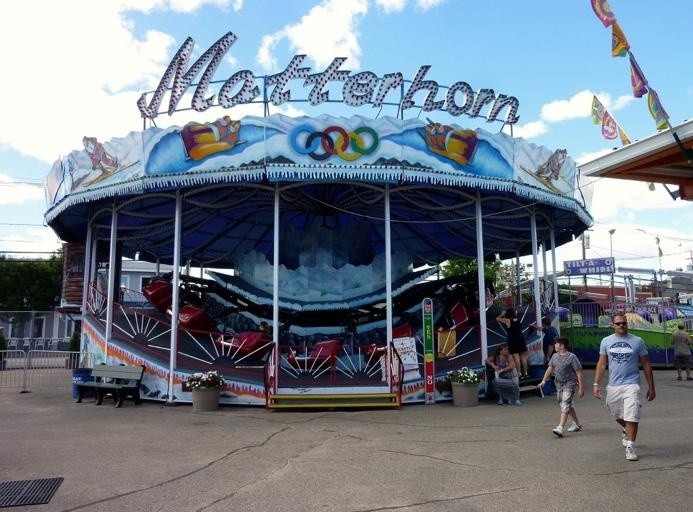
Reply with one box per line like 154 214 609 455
593 312 657 463
537 336 584 437
528 318 559 396
671 323 692 381
485 344 522 405
495 307 528 378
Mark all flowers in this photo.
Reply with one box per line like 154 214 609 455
445 365 479 383
185 369 224 391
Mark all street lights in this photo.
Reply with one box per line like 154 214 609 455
22 296 31 310
119 282 125 307
656 269 666 334
526 263 533 279
607 226 618 313
53 296 58 305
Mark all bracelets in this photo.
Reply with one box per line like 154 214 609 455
593 382 601 386
541 379 545 384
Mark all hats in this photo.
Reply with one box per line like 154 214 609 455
678 322 685 328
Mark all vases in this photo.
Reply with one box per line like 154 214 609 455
450 381 478 407
190 387 219 412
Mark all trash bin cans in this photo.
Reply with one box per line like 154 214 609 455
528 365 553 396
71 368 94 399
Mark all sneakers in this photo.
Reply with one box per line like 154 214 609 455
553 424 565 438
625 440 640 461
622 427 629 446
567 421 583 432
497 399 504 405
515 399 523 406
677 375 693 381
518 374 530 381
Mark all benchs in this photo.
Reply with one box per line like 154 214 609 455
74 362 149 406
487 364 547 401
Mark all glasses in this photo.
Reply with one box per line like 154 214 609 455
614 321 627 326
